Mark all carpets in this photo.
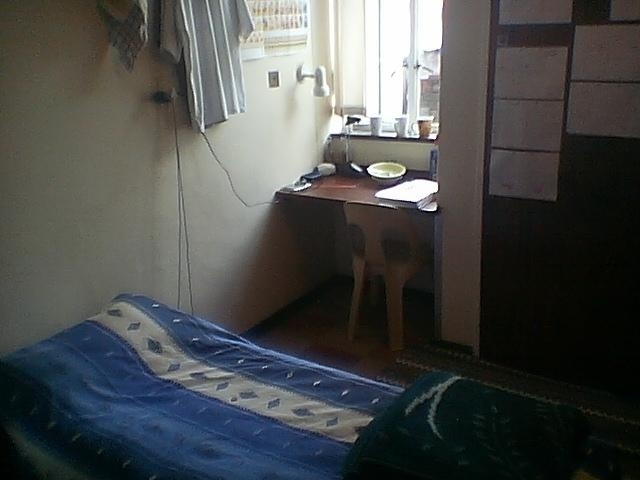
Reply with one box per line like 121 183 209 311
379 338 640 479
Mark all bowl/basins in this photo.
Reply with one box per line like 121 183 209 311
366 162 407 185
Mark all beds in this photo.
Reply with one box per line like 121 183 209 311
0 292 595 480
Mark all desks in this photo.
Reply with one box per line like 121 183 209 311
275 163 439 217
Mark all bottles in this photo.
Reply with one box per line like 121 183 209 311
329 133 349 164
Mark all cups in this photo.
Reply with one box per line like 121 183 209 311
395 117 407 138
370 117 382 136
411 117 434 139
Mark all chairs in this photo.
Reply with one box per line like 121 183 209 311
343 200 429 352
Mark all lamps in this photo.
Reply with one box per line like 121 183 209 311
297 63 329 97
